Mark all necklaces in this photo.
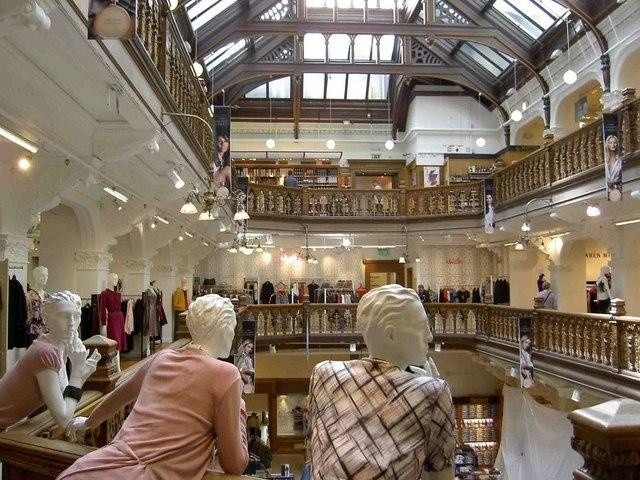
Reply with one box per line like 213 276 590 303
361 357 393 366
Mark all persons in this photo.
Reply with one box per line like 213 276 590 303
236 338 254 393
596 266 610 313
536 281 558 310
519 334 534 387
283 168 299 213
372 180 385 215
174 276 189 312
26 265 49 353
484 191 494 233
605 134 622 200
417 284 432 303
214 129 230 195
0 290 103 432
246 412 260 436
304 283 456 480
99 272 126 368
54 293 250 480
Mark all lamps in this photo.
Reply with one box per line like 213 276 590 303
288 228 325 269
326 88 336 150
515 198 552 254
399 225 420 267
189 22 205 77
179 166 251 221
384 100 395 150
475 92 486 148
17 159 31 170
341 237 352 248
586 205 603 219
218 220 274 255
167 170 184 189
563 19 578 84
511 58 522 122
266 93 276 148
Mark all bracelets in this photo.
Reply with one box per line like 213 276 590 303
62 385 84 403
